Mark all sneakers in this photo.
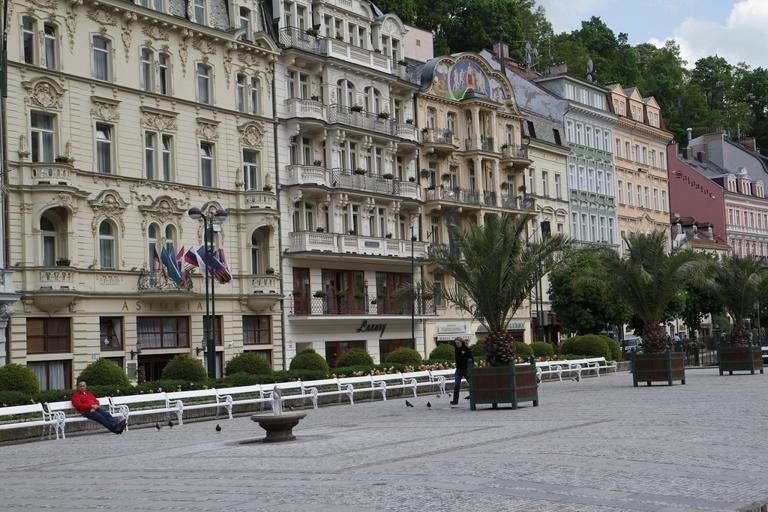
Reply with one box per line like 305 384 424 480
115 419 128 434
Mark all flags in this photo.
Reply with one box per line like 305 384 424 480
152 240 231 288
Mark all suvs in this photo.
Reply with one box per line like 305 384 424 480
618 336 642 355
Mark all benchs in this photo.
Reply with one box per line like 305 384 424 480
514 356 617 384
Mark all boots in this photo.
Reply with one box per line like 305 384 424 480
450 390 460 405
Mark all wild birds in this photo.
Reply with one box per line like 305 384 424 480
405 399 414 407
156 421 160 430
436 394 441 397
168 420 175 429
427 401 431 409
464 395 471 400
448 392 452 398
216 424 221 432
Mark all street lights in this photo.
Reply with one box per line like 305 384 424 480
188 204 229 384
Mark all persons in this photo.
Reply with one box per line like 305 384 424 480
71 378 127 434
450 336 472 405
670 332 681 351
681 335 688 351
